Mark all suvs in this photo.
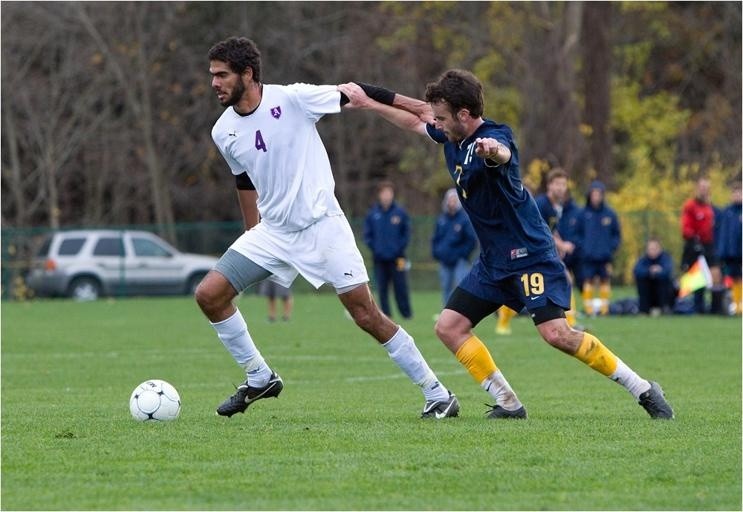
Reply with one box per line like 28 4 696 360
24 229 243 301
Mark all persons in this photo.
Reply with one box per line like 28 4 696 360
431 188 478 319
363 181 412 319
337 68 676 420
196 36 460 420
261 278 293 322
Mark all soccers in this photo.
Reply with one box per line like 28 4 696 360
131 380 181 421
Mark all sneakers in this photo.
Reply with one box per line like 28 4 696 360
638 381 673 419
487 405 526 419
216 369 283 418
421 390 461 419
